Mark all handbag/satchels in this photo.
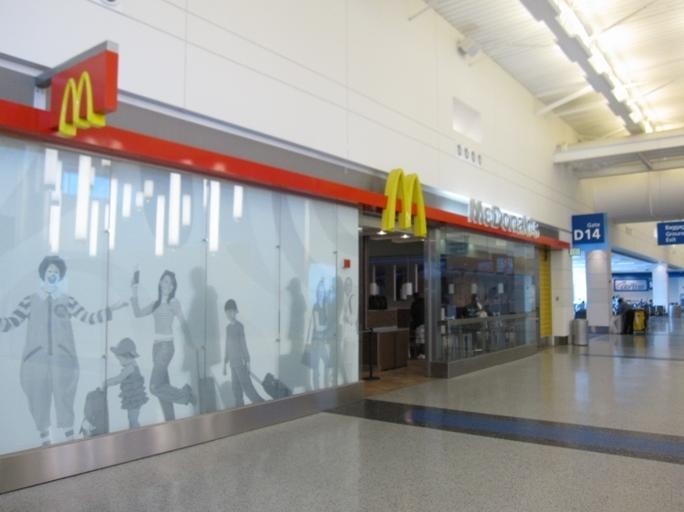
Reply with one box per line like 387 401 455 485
301 344 319 368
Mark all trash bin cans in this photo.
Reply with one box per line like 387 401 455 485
573 318 589 346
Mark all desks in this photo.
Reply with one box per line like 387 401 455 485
438 318 485 360
485 313 528 351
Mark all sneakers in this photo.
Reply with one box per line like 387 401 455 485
183 384 197 407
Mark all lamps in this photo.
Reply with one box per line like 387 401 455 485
406 260 413 295
458 37 479 57
370 264 379 296
400 264 407 300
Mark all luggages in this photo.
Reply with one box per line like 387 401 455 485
79 355 108 437
248 372 294 400
196 346 217 414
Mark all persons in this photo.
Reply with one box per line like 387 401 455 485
409 291 425 359
615 298 634 335
464 294 482 317
0 257 357 449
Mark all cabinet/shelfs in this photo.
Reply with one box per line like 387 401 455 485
363 331 409 372
